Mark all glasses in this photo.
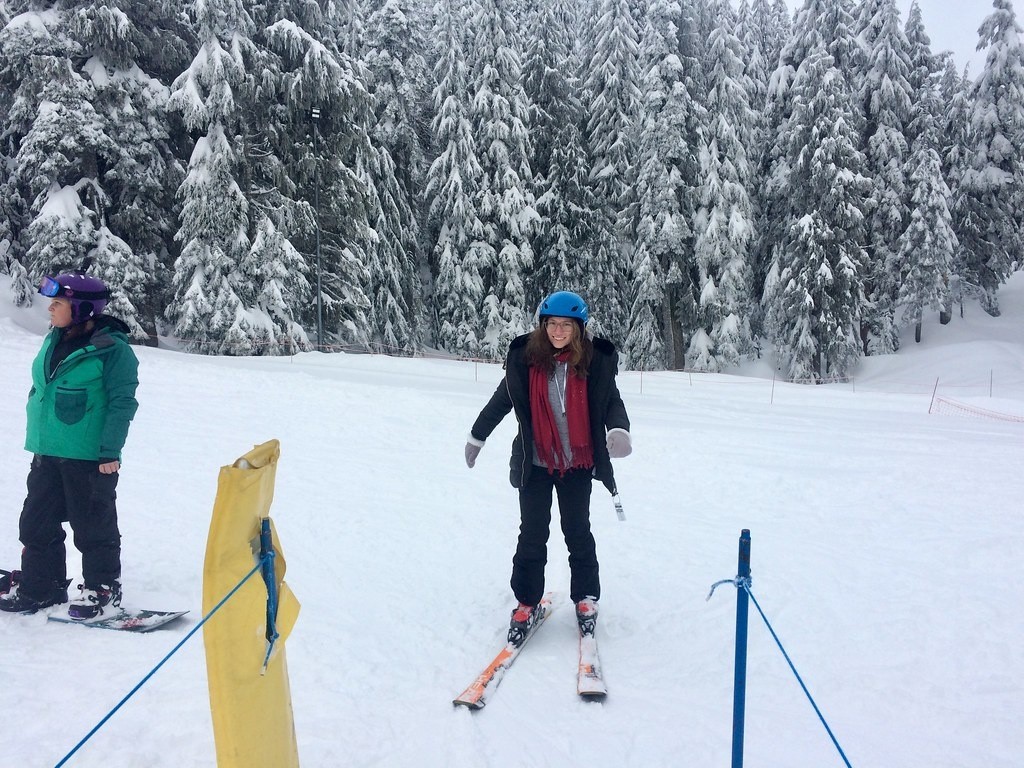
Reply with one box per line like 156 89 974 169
38 276 61 297
543 322 572 332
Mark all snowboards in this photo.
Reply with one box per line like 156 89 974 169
0 569 191 634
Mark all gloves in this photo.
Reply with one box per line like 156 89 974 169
465 433 486 468
605 428 632 458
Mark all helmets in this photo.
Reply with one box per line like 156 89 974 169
53 274 108 325
539 292 588 328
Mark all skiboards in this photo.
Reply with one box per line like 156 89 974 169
452 589 608 711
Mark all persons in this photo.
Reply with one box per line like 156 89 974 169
465 291 632 647
0 273 140 620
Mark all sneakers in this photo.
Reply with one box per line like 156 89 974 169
68 582 122 621
510 603 538 633
575 597 599 621
0 578 73 614
